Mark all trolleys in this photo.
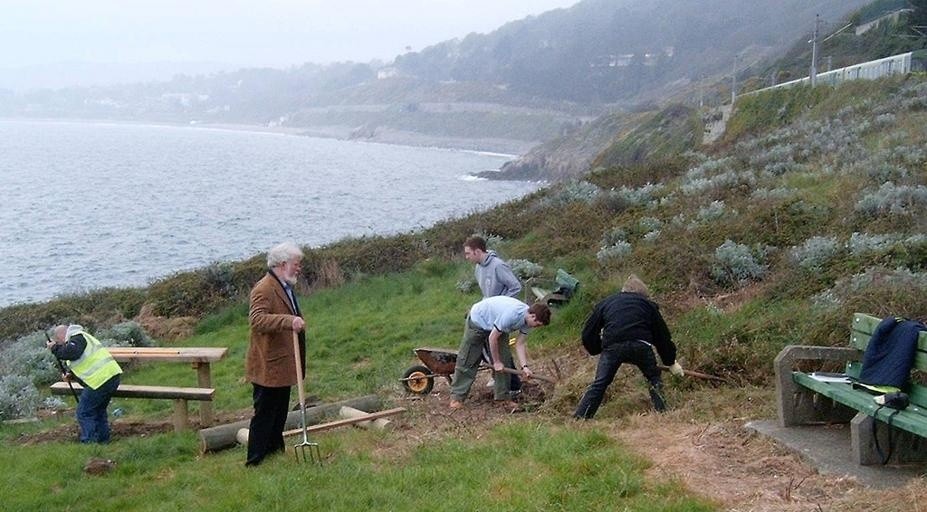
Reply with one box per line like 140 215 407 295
399 343 494 399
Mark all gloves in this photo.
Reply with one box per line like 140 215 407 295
669 360 684 378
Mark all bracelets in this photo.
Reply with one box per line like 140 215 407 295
521 364 528 370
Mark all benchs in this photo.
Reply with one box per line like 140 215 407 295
773 313 927 466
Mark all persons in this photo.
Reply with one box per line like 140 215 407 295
449 295 551 410
46 324 123 444
572 272 686 419
246 243 306 466
463 235 521 394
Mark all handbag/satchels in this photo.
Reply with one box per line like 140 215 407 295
873 390 909 409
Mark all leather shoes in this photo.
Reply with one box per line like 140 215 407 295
450 400 464 410
495 401 523 411
